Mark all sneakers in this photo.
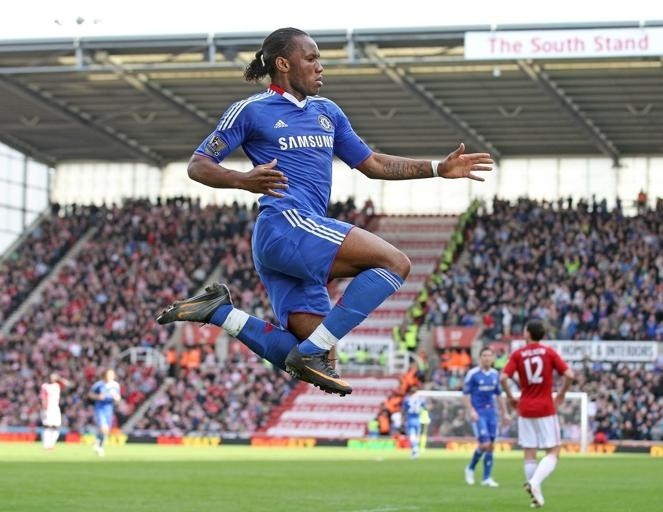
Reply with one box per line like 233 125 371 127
155 283 233 325
465 466 475 485
524 481 544 507
283 344 353 396
480 478 498 488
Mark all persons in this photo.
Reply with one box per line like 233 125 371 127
153 28 494 396
87 367 122 456
1 195 376 435
462 346 498 486
499 321 575 509
368 190 663 459
37 372 75 454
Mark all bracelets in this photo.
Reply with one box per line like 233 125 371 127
431 160 440 177
